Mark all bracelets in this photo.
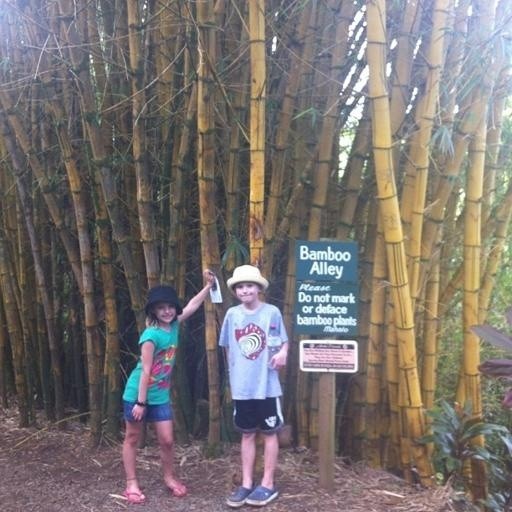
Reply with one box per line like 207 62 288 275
136 402 146 407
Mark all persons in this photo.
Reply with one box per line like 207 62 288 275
121 269 217 506
218 264 290 509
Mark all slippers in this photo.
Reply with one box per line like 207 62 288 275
172 482 187 497
123 488 146 503
226 485 279 508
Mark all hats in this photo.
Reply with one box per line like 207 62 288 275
226 265 269 296
145 286 183 315
